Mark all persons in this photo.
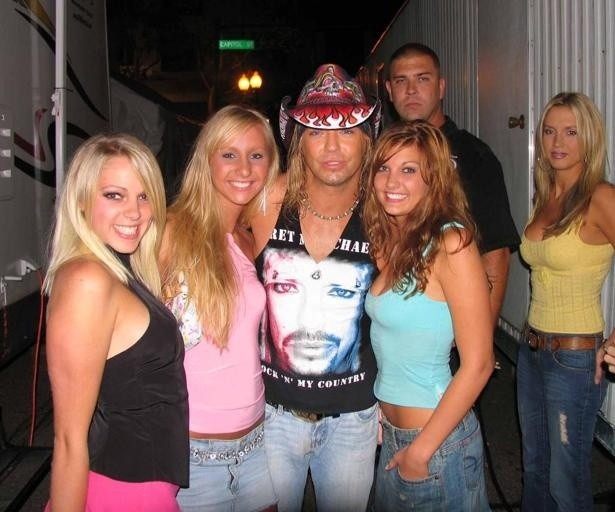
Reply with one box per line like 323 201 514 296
260 246 375 378
382 44 518 428
160 105 281 512
44 133 190 512
359 120 493 509
516 91 613 512
242 63 395 512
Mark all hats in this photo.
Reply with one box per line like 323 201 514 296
275 63 382 142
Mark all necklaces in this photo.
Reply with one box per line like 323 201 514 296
297 189 363 223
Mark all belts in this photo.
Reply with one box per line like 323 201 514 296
265 397 342 422
521 321 594 352
